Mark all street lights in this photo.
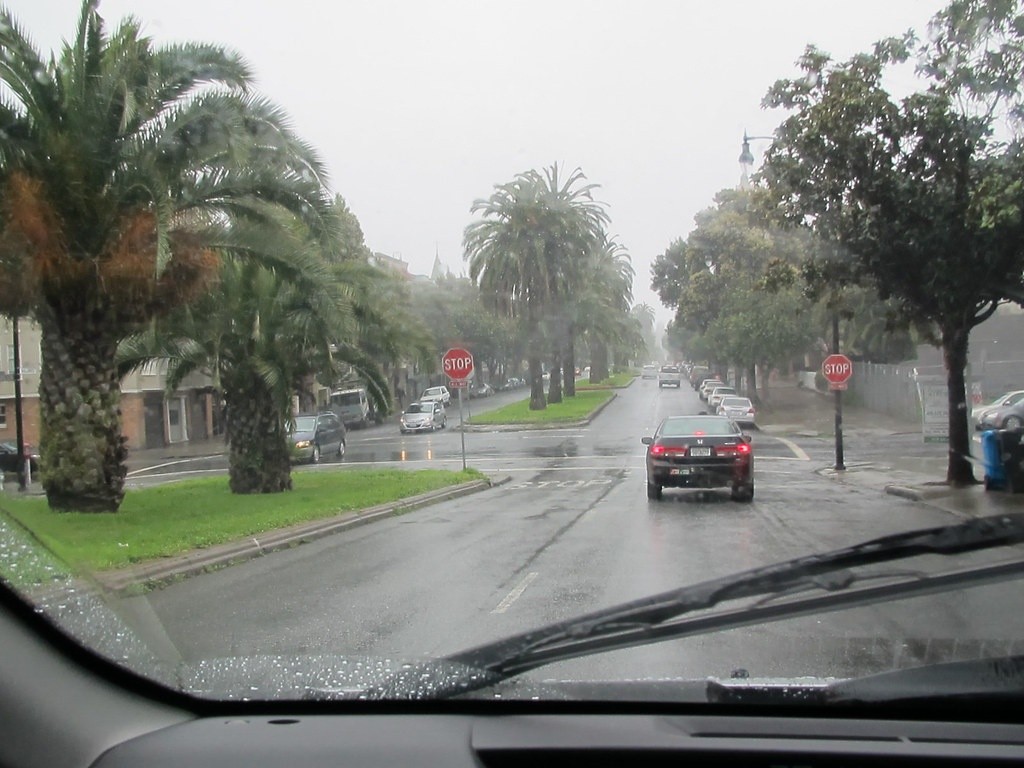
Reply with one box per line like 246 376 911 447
737 130 847 470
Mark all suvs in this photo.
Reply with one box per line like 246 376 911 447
659 365 680 387
281 411 348 463
689 367 709 385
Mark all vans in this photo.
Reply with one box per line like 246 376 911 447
329 388 370 427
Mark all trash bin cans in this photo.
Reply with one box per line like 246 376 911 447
978 426 1024 494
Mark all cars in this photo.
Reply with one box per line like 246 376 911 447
575 368 581 376
715 398 755 427
970 391 1024 430
471 384 495 398
699 379 723 400
641 365 658 378
641 411 755 502
682 361 694 378
707 388 737 408
504 377 524 388
400 403 446 434
0 442 38 475
542 371 551 380
421 386 450 406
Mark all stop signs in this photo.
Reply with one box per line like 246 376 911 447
442 347 473 381
821 354 852 385
